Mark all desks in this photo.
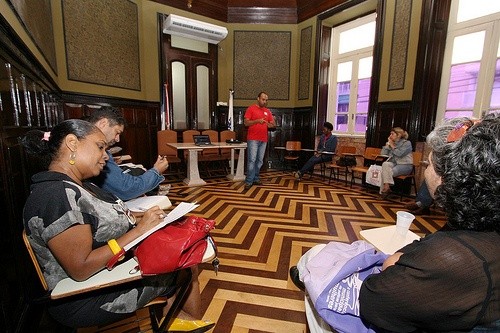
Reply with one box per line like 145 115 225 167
342 152 363 188
299 148 318 177
51 234 216 333
319 151 337 180
167 138 248 187
274 147 292 172
365 164 388 195
358 222 423 263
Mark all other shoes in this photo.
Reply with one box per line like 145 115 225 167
289 266 305 291
158 184 171 196
381 189 392 193
407 204 430 216
244 184 251 190
376 195 389 200
253 182 263 185
293 172 303 181
159 317 215 333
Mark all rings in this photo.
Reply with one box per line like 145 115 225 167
159 214 164 219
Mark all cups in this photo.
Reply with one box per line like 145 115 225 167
395 211 415 235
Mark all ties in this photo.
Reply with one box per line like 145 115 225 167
321 132 332 144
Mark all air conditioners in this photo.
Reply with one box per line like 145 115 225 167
162 14 229 45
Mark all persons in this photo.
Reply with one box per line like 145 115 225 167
289 112 500 333
22 119 215 332
294 122 337 182
378 127 414 200
244 92 275 190
407 179 433 215
88 107 169 196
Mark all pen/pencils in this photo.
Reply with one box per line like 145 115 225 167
135 207 150 211
161 154 165 161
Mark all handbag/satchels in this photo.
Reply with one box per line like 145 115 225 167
336 157 357 166
365 164 382 187
133 215 215 277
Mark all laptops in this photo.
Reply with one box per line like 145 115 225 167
193 135 220 146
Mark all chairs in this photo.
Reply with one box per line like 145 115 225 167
286 142 423 203
157 130 245 182
22 210 158 333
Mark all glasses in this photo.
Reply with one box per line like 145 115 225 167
419 158 433 171
116 199 136 225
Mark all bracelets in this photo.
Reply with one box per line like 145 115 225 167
107 239 125 262
